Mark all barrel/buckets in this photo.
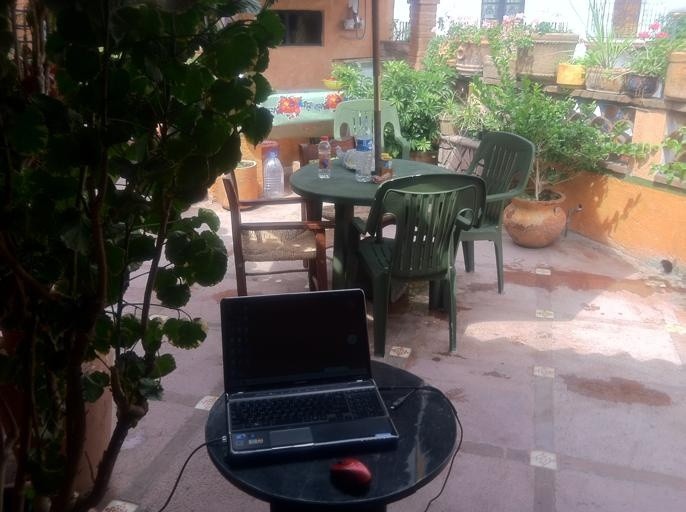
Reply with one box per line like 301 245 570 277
455 42 482 78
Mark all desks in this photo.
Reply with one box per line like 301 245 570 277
288 156 461 311
202 355 461 510
243 86 348 190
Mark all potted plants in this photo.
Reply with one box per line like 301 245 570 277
429 0 686 144
320 62 357 91
482 79 659 247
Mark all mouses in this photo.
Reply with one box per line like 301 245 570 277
330 458 371 484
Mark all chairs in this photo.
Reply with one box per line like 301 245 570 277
346 173 487 357
299 136 401 268
461 132 537 291
224 166 333 295
334 98 410 159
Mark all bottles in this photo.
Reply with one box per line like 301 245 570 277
317 136 333 179
355 119 373 182
340 148 378 171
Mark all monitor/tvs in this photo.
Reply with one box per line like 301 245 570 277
269 11 322 43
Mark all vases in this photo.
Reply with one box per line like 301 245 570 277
214 158 260 211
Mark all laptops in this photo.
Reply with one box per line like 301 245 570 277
220 288 400 457
